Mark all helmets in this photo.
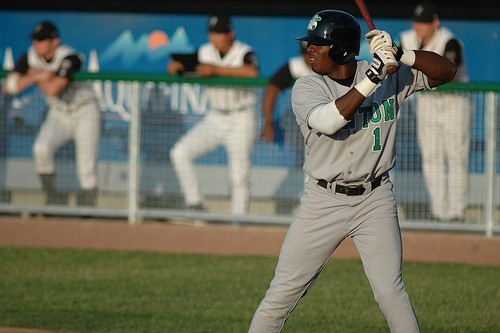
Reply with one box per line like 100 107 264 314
294 9 361 65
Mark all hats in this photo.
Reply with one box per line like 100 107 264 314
208 14 232 34
31 21 58 40
411 3 438 22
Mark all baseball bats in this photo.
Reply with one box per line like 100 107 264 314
356 0 397 74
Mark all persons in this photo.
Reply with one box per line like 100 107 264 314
164 12 260 228
258 32 323 145
250 8 459 333
392 2 473 225
0 18 102 207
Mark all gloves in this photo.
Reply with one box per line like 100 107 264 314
364 48 399 85
365 29 404 62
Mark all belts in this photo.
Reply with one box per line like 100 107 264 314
317 175 382 197
